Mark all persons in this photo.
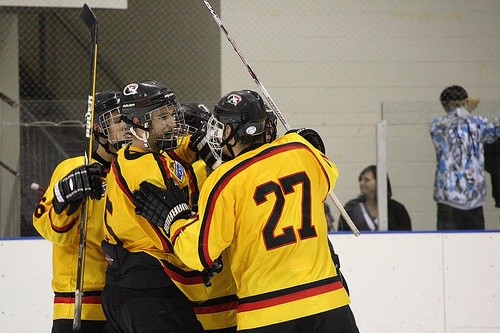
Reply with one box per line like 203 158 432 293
430 86 500 231
134 89 359 333
337 165 412 233
101 81 238 333
33 91 134 333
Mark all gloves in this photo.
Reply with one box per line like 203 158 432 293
51 164 107 216
132 180 191 238
201 253 223 287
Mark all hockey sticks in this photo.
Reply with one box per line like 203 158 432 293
202 0 360 238
72 3 101 331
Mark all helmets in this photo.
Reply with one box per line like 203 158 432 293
120 80 177 130
263 103 277 143
214 89 267 138
93 88 122 135
177 102 213 134
